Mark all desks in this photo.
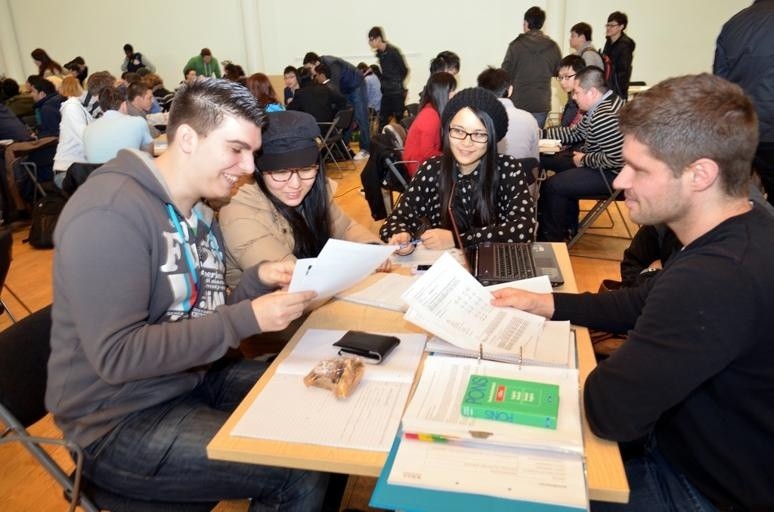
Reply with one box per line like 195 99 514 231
204 241 632 512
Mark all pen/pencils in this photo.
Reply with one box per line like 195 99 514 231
400 240 422 249
305 265 312 275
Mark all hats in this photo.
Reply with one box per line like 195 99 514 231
442 88 508 142
257 110 320 169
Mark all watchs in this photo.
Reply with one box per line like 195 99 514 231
640 266 659 275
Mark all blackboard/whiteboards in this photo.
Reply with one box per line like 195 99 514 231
561 1 756 91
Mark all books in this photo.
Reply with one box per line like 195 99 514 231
461 372 560 431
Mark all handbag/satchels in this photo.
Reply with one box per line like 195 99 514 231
30 197 67 250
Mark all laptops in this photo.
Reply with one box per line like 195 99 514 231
448 181 564 287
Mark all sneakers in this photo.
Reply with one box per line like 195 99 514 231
353 150 370 160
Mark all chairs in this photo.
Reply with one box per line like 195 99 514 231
1 305 221 512
0 76 773 320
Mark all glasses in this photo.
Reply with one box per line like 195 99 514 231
605 23 620 28
267 169 316 181
556 73 577 81
450 124 488 142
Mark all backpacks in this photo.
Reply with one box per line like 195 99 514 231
581 48 612 84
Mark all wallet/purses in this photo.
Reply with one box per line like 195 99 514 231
334 331 400 364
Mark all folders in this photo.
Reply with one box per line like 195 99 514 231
370 327 592 512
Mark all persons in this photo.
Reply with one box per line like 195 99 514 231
487 69 774 511
709 0 774 206
0 6 636 243
377 85 539 257
214 108 393 345
616 221 684 281
39 73 334 511
